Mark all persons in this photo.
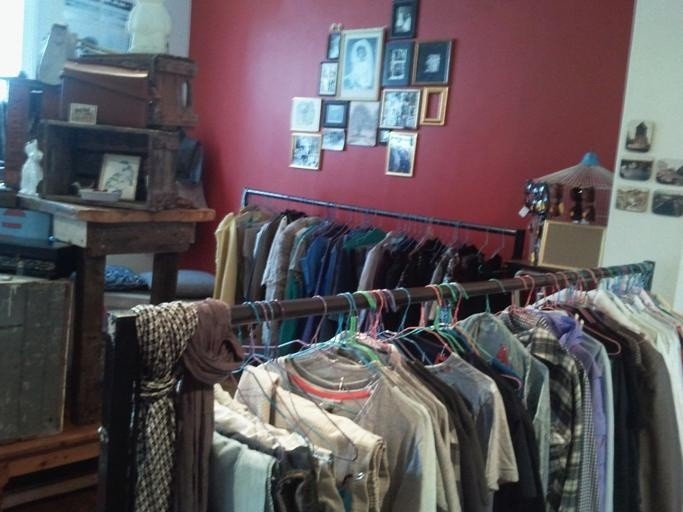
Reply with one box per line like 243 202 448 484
343 44 374 88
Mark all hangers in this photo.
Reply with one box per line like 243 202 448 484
214 256 682 464
232 185 508 267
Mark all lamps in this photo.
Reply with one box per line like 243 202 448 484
536 150 617 228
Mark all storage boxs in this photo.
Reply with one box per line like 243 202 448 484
36 49 207 211
0 270 76 447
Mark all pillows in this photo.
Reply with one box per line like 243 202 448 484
100 257 151 292
138 266 216 300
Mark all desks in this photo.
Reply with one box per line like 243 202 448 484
15 189 218 430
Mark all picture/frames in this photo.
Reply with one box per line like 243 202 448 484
287 1 454 179
